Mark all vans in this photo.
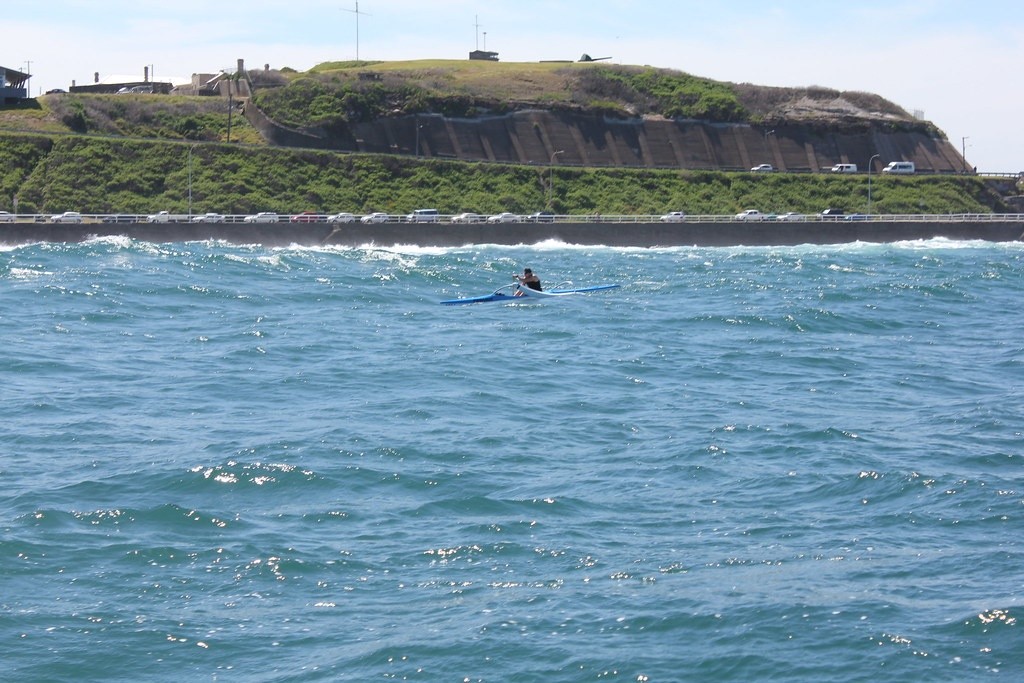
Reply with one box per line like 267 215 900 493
244 212 279 224
407 209 439 223
883 161 915 174
831 163 858 174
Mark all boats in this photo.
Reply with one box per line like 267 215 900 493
440 282 620 305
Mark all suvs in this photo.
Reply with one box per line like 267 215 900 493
51 211 85 223
816 209 844 222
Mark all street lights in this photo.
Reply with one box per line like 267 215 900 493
188 142 203 223
963 136 971 173
550 150 566 216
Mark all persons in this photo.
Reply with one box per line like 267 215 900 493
513 268 542 296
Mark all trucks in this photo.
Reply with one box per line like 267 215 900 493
146 211 189 223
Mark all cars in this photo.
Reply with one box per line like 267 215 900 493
451 213 480 224
846 213 869 221
359 213 390 225
777 212 806 222
192 213 226 223
487 213 518 223
0 211 17 222
116 87 131 96
45 88 67 96
660 211 687 223
751 164 773 172
735 210 764 223
289 211 318 223
103 214 139 223
33 214 48 223
130 86 153 95
525 211 554 223
326 212 355 224
